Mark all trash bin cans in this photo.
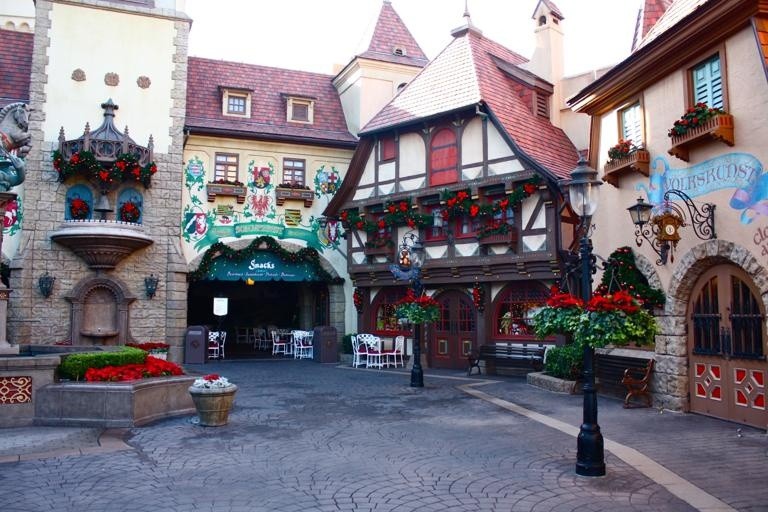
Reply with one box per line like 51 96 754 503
314 326 337 363
185 325 208 364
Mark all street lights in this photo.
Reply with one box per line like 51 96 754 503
407 237 428 389
558 157 603 479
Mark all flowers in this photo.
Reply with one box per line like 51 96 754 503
396 290 440 325
192 374 232 389
533 286 658 349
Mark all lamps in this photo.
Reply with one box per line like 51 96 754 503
627 196 667 266
145 273 159 299
39 272 55 298
652 189 716 263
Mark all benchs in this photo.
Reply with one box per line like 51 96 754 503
467 344 546 376
570 353 654 408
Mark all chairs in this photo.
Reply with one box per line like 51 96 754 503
253 327 314 360
207 331 226 359
351 334 405 369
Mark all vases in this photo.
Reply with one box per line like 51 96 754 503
188 385 239 426
